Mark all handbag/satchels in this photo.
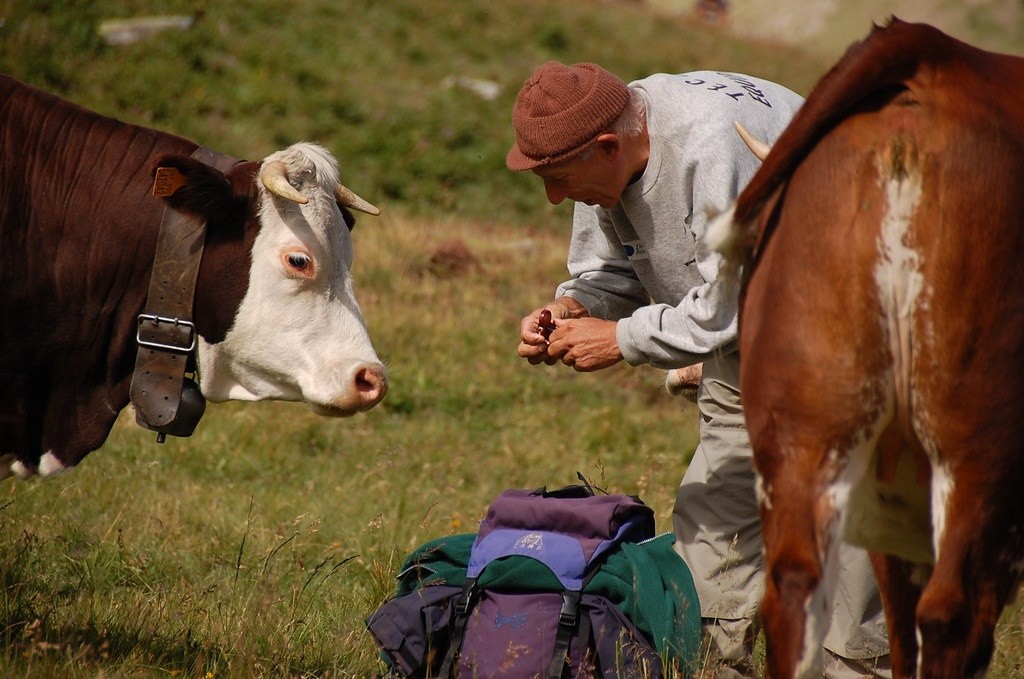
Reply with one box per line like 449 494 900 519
437 481 654 679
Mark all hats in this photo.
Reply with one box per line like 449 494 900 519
505 61 630 173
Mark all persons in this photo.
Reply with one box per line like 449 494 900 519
506 62 891 679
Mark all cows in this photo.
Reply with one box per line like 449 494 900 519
0 77 389 480
695 16 1024 678
